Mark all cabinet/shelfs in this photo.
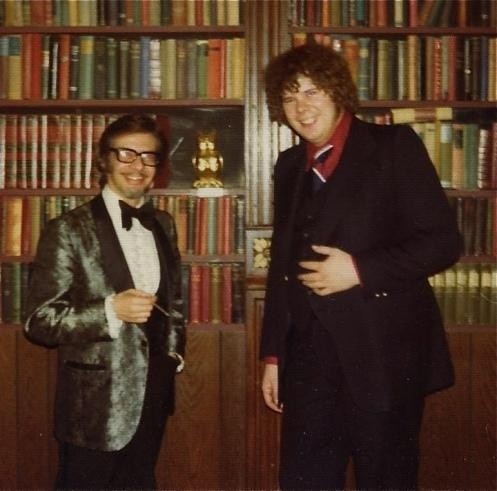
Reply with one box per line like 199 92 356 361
0 0 497 491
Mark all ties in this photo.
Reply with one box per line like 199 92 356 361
311 143 333 194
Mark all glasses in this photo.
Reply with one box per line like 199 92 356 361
109 148 160 167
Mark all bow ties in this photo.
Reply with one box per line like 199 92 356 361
119 199 154 231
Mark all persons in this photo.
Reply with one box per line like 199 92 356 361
22 114 186 491
261 48 462 490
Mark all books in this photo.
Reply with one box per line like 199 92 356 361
287 1 497 329
0 1 244 324
